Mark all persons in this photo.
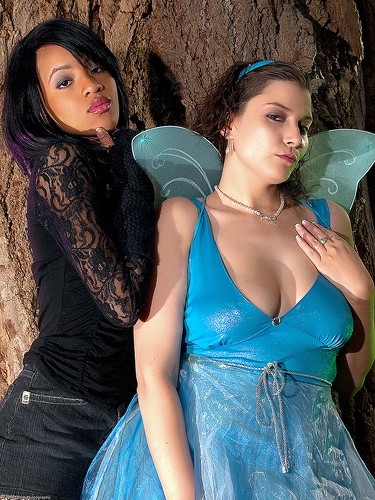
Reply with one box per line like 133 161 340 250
1 17 155 500
82 59 375 500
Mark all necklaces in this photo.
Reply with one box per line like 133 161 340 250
214 183 284 222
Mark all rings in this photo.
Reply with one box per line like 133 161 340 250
321 237 328 244
